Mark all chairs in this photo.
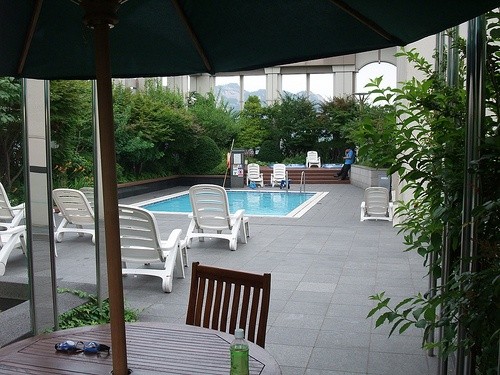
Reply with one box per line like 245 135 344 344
0 181 28 277
184 184 249 251
186 261 271 348
306 151 321 168
360 186 393 221
271 164 286 187
117 203 190 292
246 163 264 187
53 188 96 243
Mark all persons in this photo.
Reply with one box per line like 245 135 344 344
333 145 354 181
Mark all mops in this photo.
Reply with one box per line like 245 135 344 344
221 138 235 191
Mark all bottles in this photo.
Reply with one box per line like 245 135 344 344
229 328 249 375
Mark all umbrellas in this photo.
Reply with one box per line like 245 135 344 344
1 0 500 375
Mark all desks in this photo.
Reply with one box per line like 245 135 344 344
0 320 284 375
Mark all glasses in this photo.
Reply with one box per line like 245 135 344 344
55 340 111 355
